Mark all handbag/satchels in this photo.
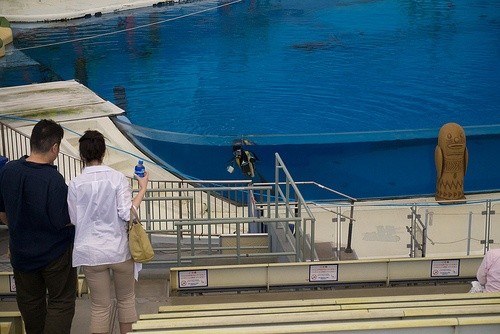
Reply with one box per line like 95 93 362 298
128 205 155 264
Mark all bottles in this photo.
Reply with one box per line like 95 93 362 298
134 160 145 180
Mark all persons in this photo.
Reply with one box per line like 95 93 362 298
227 145 260 177
467 248 500 292
66 130 148 334
0 119 76 334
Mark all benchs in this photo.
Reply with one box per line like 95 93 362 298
0 271 89 334
126 292 500 334
167 256 484 297
